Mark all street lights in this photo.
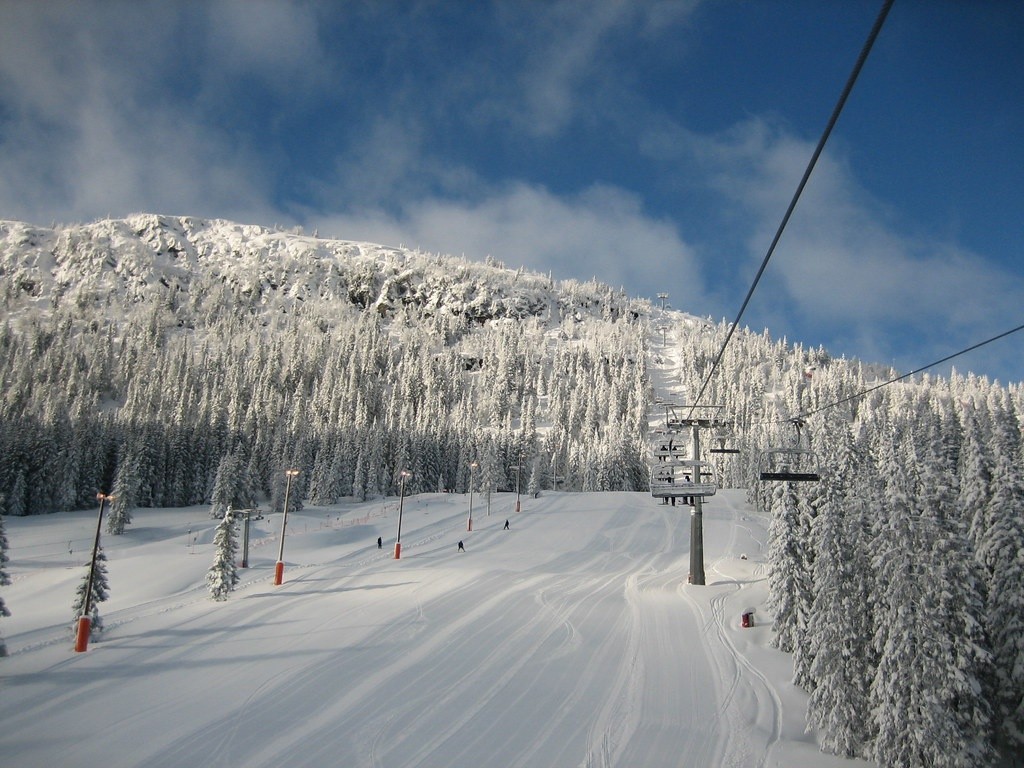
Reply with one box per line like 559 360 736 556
394 470 412 558
273 470 302 585
468 463 480 531
516 453 524 512
72 494 117 651
